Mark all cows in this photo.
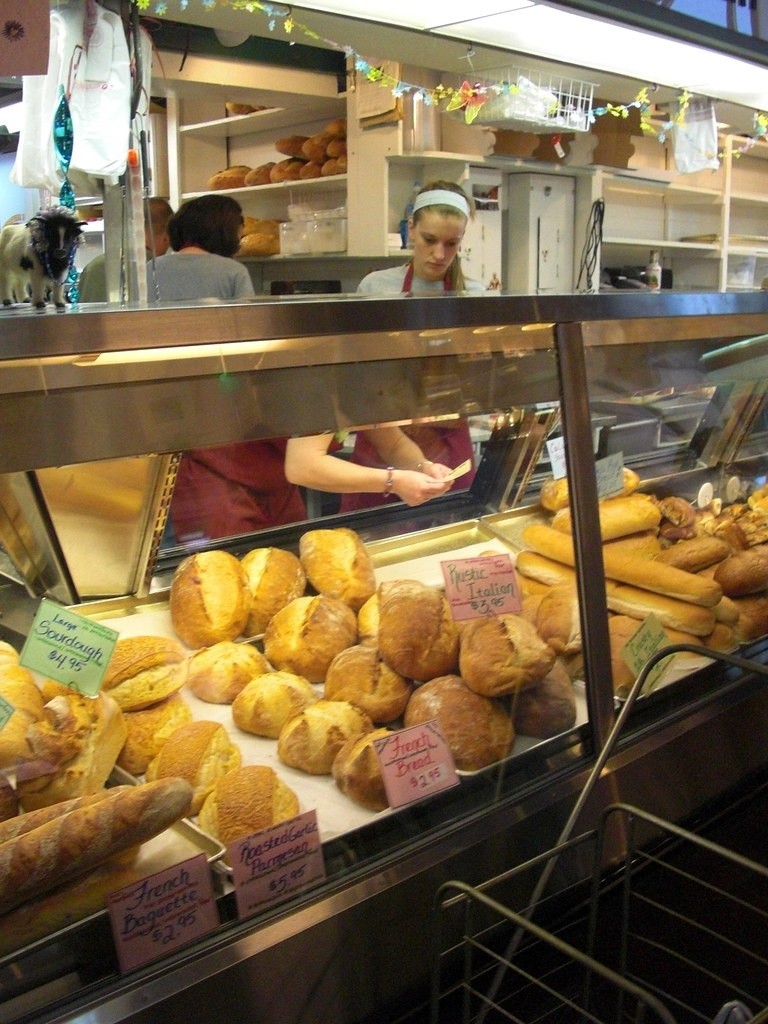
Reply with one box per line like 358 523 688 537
0 205 89 314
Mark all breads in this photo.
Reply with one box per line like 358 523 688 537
0 527 644 970
206 101 347 257
478 468 768 699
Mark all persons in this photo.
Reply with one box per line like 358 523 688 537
340 179 476 514
77 197 174 303
146 194 256 301
171 426 455 544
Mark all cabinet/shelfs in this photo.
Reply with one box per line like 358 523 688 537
166 97 348 261
384 116 767 292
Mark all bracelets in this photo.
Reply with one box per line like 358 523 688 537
383 467 393 498
415 461 432 472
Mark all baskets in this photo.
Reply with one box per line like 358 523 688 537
457 66 600 133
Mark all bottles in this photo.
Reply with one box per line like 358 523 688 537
645 250 661 291
